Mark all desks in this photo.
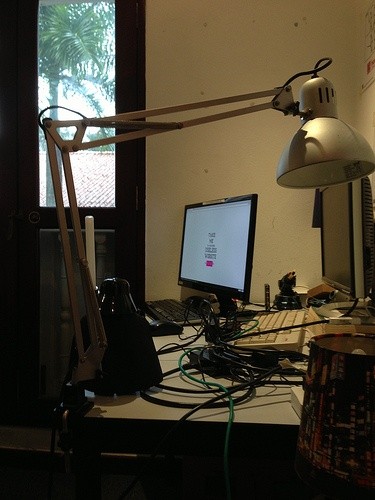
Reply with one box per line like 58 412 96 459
83 296 346 500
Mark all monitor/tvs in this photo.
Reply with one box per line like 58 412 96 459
318 174 375 317
177 193 258 316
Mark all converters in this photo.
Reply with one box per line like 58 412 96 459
190 349 241 378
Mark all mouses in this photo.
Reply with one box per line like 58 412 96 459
149 321 183 336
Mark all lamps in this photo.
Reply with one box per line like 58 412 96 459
36 57 375 389
298 332 375 500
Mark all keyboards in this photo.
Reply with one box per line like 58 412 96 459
145 299 205 325
229 309 307 354
